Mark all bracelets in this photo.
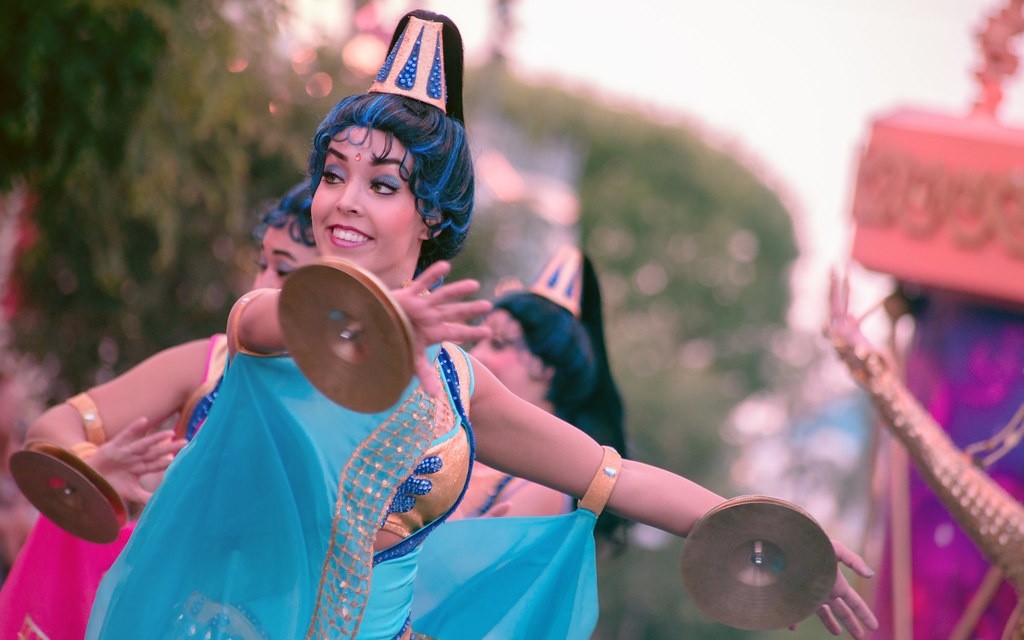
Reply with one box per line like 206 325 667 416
71 441 98 459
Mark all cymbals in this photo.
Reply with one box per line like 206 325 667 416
275 258 419 416
677 492 840 632
7 435 130 546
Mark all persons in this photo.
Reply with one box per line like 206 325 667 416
83 9 879 640
445 244 637 559
0 176 322 640
